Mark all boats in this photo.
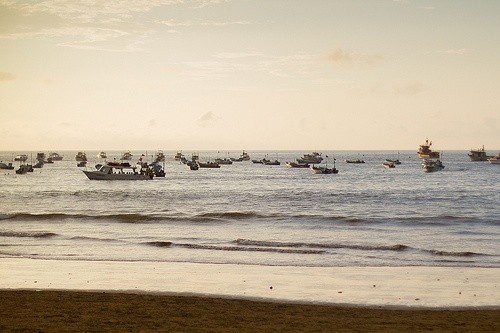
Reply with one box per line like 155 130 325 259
311 159 338 175
345 159 365 163
297 151 328 163
252 153 280 165
0 152 63 174
468 145 494 161
422 158 445 172
487 153 500 164
285 159 310 168
175 148 250 170
74 150 165 180
382 159 401 168
417 139 440 160
